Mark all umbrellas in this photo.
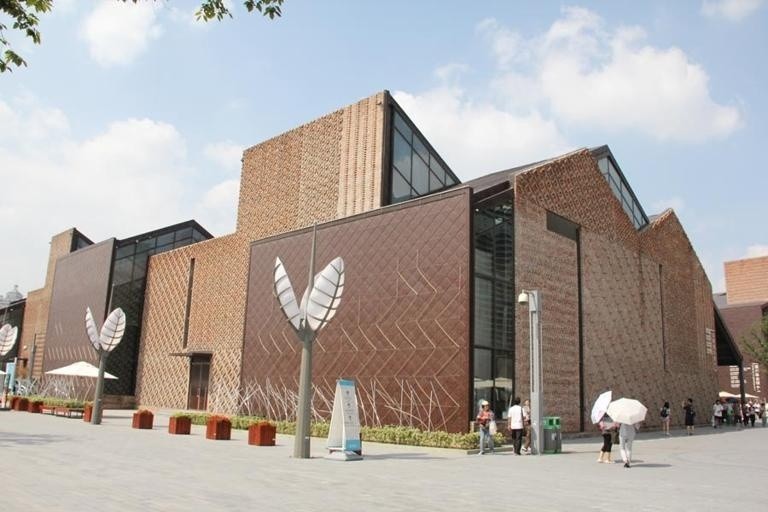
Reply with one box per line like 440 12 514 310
44 360 119 402
607 398 648 426
591 390 612 425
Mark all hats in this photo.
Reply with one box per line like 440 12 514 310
482 401 489 405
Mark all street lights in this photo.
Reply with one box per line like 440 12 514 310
515 286 547 456
83 281 127 426
0 306 19 357
271 219 346 459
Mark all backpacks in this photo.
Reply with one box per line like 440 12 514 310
660 407 668 417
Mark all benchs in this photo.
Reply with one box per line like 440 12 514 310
39 405 84 418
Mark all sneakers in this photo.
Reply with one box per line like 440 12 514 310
624 464 630 467
598 460 615 464
513 448 532 455
478 449 494 456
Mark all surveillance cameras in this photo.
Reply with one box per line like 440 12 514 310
518 289 529 305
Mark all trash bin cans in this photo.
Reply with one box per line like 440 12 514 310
542 416 562 454
727 408 735 425
761 411 768 428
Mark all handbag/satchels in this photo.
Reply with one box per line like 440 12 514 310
488 420 497 435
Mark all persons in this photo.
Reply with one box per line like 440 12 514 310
476 401 495 455
508 397 530 455
682 398 696 434
713 400 768 428
660 402 672 436
598 414 640 468
